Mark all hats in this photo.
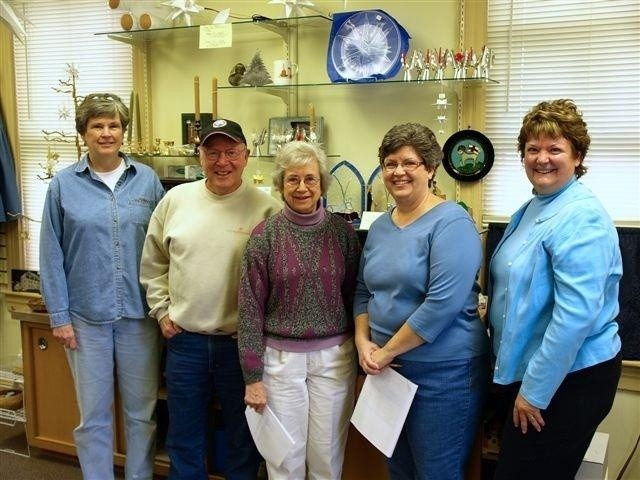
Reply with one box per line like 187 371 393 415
200 119 246 145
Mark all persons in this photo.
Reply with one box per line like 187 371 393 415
39 92 166 480
484 99 624 480
236 140 364 480
139 119 285 480
352 122 492 480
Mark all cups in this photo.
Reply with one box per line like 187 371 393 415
399 46 495 83
273 59 299 86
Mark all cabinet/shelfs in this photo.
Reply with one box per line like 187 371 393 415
19 321 483 480
90 14 500 159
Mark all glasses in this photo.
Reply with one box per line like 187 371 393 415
383 159 425 172
286 174 320 189
88 94 116 102
201 148 244 161
